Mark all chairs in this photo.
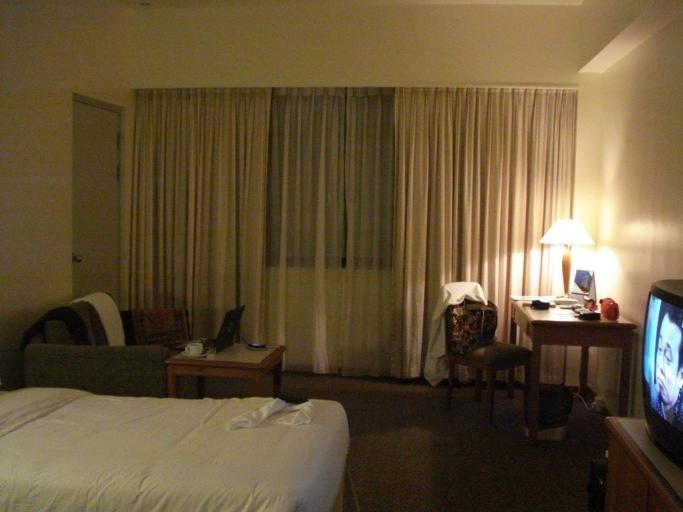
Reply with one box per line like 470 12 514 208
24 291 189 398
441 281 531 430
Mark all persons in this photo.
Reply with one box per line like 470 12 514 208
648 306 682 426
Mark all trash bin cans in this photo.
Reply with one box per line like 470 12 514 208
523 383 572 440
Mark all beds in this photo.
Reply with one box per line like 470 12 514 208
0 387 350 511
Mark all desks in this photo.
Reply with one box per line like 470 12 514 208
507 296 638 447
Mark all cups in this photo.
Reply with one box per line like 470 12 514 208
184 342 203 355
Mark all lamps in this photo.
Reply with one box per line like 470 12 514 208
538 219 596 295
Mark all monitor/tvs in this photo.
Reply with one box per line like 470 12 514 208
642 279 683 472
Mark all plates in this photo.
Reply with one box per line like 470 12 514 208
180 351 206 358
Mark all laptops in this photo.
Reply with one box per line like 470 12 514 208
175 306 246 351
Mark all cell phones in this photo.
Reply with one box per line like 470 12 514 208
574 312 600 320
